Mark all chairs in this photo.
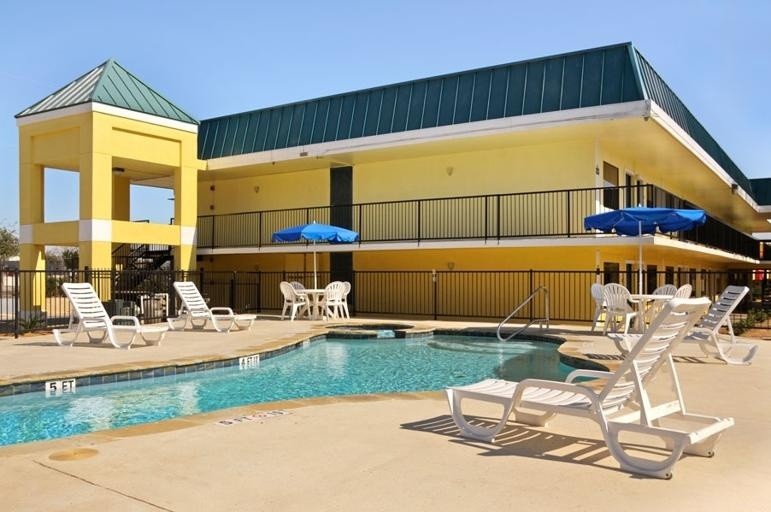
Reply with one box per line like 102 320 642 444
166 281 259 334
50 281 171 351
277 281 352 323
444 294 737 480
588 279 694 337
605 284 759 367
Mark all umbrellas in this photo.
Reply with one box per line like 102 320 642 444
272 220 359 289
583 203 706 294
